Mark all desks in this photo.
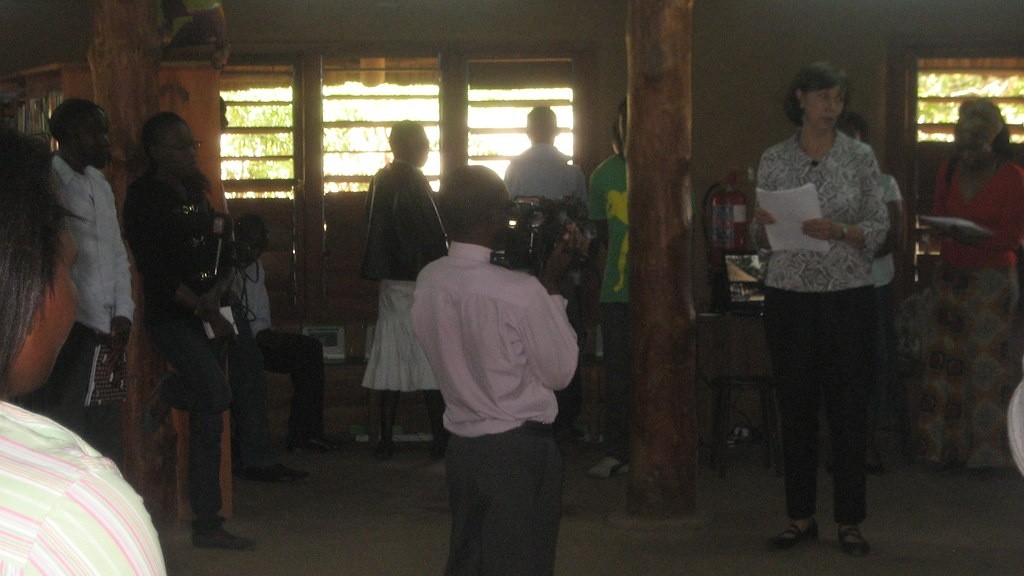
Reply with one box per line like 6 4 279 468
695 312 908 470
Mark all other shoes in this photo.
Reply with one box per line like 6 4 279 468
240 462 309 483
430 442 446 457
288 436 340 453
192 528 255 551
373 444 393 460
838 523 870 555
765 518 819 551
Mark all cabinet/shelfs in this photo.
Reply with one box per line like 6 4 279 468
0 61 94 152
157 60 235 522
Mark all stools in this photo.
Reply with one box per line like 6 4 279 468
705 374 785 480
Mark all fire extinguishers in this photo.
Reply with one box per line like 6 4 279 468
702 170 749 284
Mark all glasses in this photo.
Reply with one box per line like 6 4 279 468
160 140 202 151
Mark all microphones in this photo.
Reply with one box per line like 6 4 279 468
538 199 588 221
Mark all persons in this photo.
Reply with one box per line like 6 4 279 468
410 165 584 575
750 66 905 554
51 97 135 465
502 98 700 476
364 121 449 457
122 112 254 551
0 129 167 576
226 201 338 453
928 98 1024 485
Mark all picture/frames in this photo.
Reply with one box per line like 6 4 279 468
301 322 348 364
